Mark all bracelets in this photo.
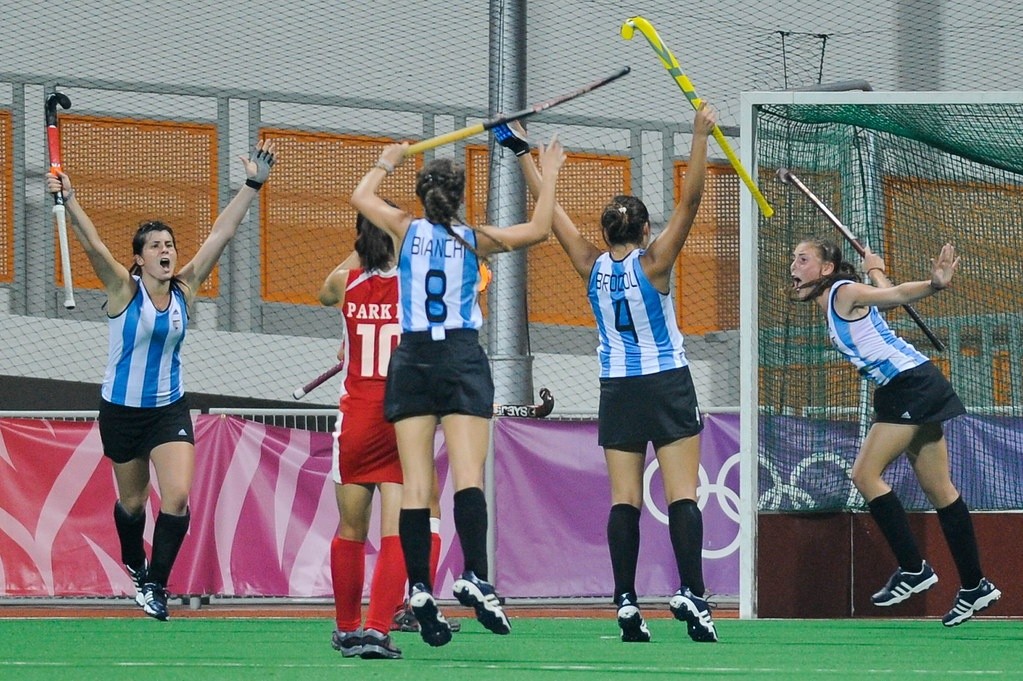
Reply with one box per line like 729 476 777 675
867 267 885 275
374 159 393 173
930 280 944 290
63 188 73 202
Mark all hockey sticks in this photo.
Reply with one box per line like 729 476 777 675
775 168 948 353
292 260 494 400
404 65 631 158
620 14 774 218
44 91 72 310
493 387 554 419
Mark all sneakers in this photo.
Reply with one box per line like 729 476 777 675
135 578 171 619
616 592 651 642
871 559 939 607
409 581 452 646
396 598 461 633
668 586 720 642
942 577 1002 627
331 629 363 658
387 598 410 629
127 558 150 606
452 569 512 635
361 630 402 659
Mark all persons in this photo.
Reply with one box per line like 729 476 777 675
789 234 1002 626
349 133 567 647
491 100 718 642
45 139 279 621
319 200 461 658
